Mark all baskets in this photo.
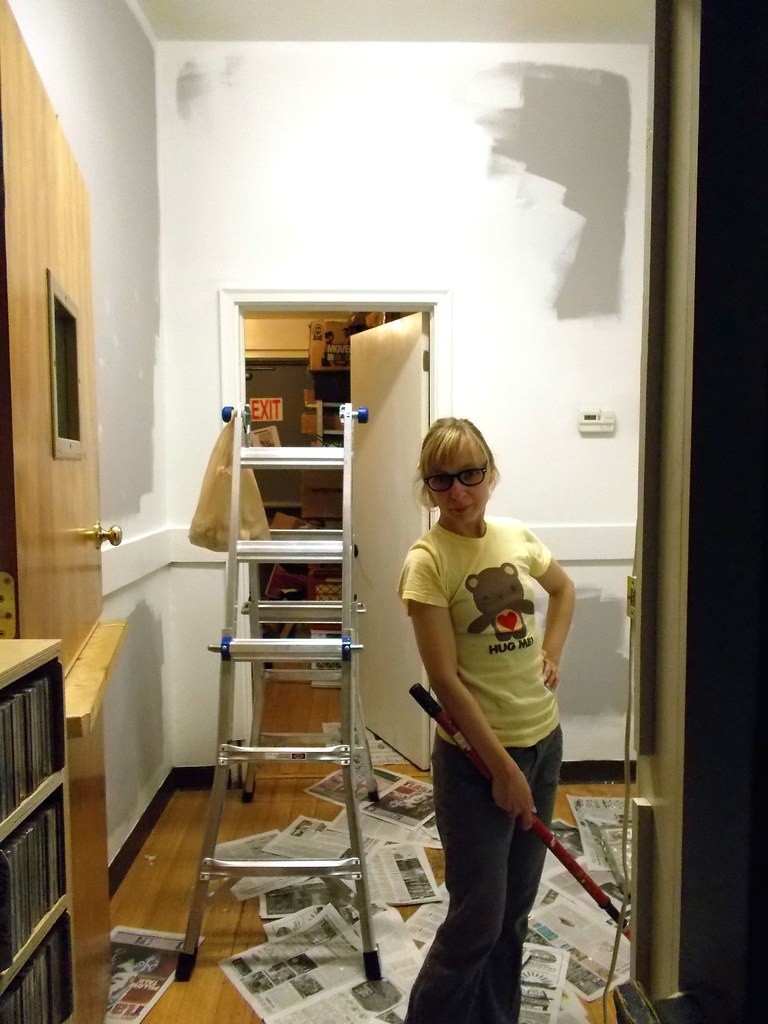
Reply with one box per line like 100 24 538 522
307 575 344 630
311 631 343 688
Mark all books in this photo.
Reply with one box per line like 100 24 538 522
0 667 73 1024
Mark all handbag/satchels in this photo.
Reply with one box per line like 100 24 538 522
188 409 274 552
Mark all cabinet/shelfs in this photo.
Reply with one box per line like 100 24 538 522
1 638 79 1024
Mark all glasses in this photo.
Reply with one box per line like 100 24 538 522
423 458 488 491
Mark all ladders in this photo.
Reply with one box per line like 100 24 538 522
175 403 383 986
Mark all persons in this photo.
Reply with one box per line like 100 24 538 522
397 416 578 1024
